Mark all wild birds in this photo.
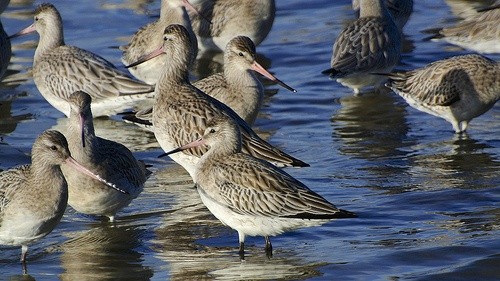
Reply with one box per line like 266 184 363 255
120 0 358 258
0 91 153 281
323 1 403 96
7 2 153 118
428 7 500 53
369 53 500 132
